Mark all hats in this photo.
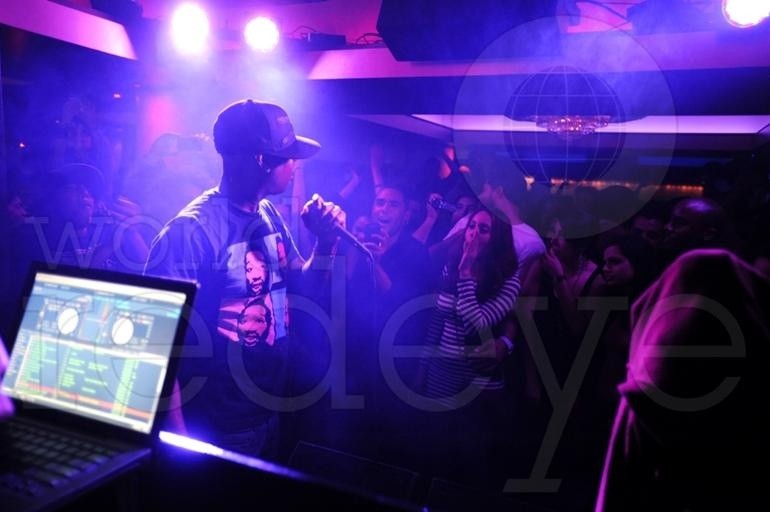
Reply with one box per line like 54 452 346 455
213 99 321 159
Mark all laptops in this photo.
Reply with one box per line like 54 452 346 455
1 260 196 512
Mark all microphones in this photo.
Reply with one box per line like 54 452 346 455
305 200 377 255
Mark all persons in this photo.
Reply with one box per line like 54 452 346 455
0 98 770 512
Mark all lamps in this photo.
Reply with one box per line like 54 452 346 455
720 0 770 29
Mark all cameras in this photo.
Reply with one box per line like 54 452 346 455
430 198 456 214
